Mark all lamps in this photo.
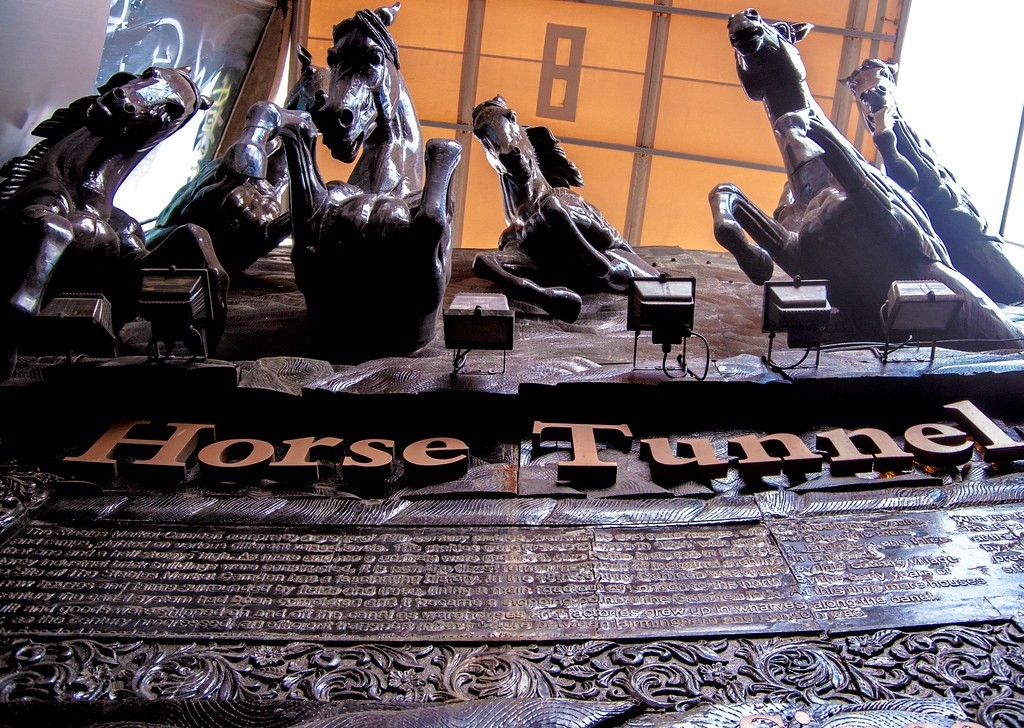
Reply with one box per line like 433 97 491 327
139 266 215 360
626 275 697 370
34 291 120 359
761 276 831 372
878 278 967 365
442 292 515 375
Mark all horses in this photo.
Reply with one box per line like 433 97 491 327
0 3 664 383
707 7 1024 349
